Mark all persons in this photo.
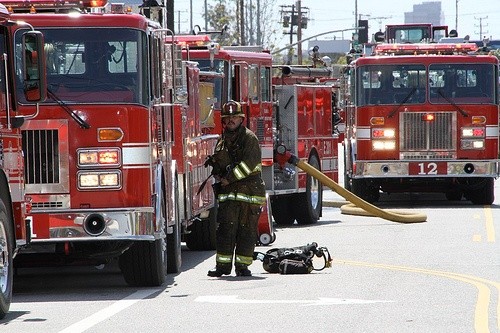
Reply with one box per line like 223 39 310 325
207 100 267 277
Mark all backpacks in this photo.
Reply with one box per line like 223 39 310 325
261 242 333 276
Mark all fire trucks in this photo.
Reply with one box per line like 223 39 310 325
0 0 343 318
344 23 500 204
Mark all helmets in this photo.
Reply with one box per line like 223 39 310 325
219 100 246 118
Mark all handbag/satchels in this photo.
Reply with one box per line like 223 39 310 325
206 149 236 178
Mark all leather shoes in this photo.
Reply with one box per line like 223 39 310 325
207 263 233 276
234 263 252 277
